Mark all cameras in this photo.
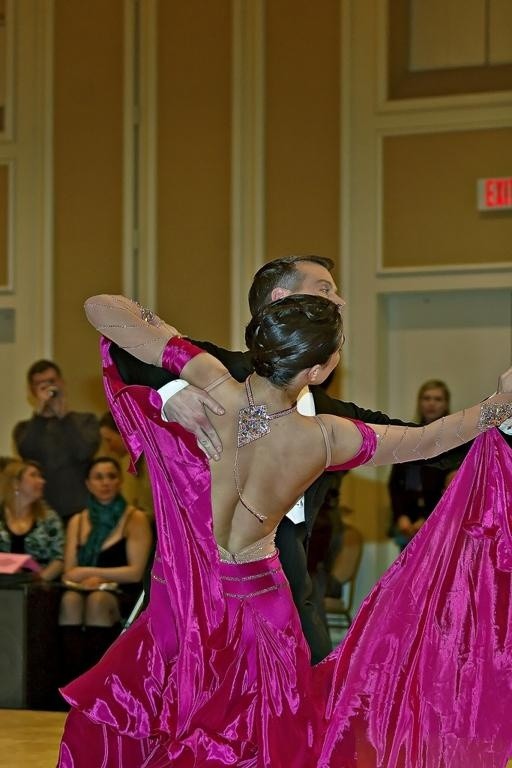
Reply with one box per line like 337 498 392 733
47 386 59 400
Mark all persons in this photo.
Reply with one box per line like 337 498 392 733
389 380 454 552
109 254 512 662
58 290 512 768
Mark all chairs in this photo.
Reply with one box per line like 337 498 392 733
324 522 365 642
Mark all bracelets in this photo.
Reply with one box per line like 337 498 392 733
478 397 510 434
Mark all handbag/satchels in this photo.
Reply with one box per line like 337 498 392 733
0 568 49 585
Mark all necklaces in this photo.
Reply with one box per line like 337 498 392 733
236 374 298 450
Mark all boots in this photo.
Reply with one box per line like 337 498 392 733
52 624 114 712
